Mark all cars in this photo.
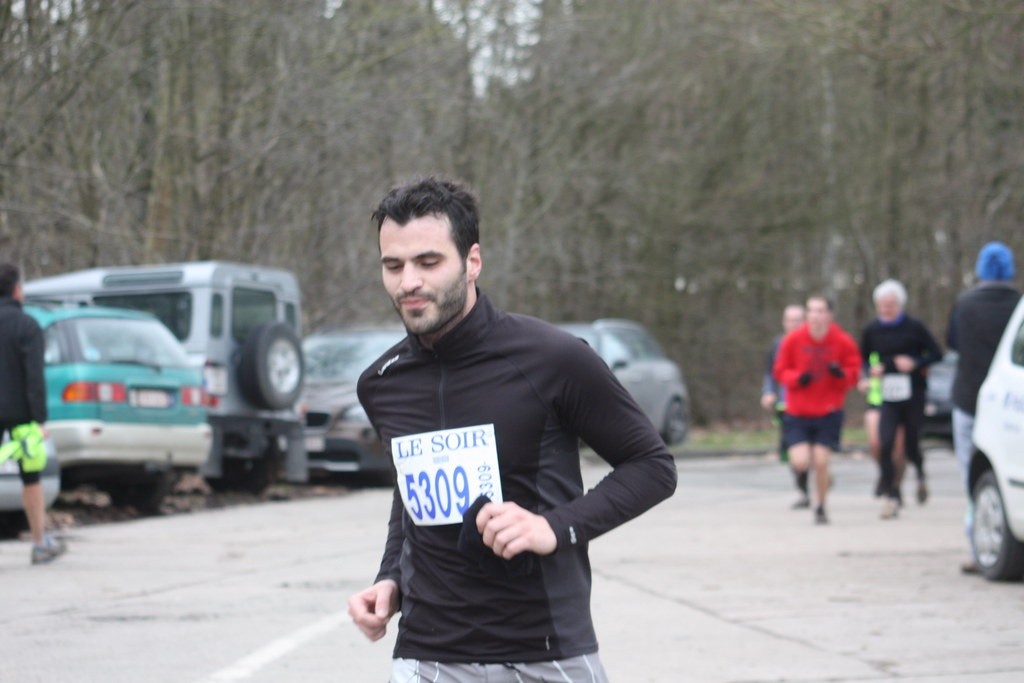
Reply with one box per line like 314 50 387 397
923 361 954 437
299 327 407 485
0 425 63 517
966 293 1024 580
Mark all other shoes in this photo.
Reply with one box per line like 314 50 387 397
916 479 926 505
879 497 898 519
816 511 826 523
795 501 807 508
34 539 63 562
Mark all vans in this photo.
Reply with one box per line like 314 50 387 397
17 260 307 514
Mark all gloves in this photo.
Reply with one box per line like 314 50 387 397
799 372 812 384
828 363 844 379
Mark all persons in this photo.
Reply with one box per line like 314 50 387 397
0 263 67 562
761 242 1020 525
350 177 678 683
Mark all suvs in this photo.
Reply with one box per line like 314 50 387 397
552 319 689 443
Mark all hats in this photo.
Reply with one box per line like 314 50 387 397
976 242 1015 280
